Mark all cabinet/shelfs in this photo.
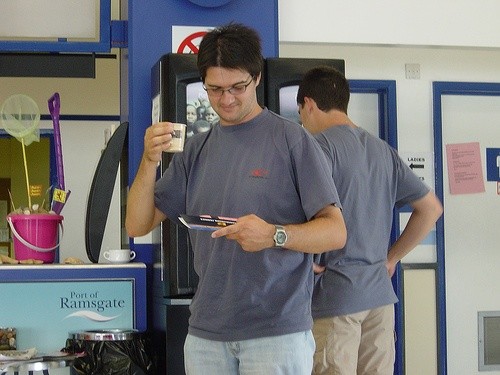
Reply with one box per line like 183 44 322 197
147 53 346 375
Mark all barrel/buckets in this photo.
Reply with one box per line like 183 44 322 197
6 213 65 264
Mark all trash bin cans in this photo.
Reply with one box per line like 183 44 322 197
68 329 165 375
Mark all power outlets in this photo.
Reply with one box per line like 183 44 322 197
406 63 422 80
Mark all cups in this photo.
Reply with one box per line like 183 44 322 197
162 123 187 153
103 250 135 264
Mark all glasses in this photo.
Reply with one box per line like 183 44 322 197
202 72 258 97
